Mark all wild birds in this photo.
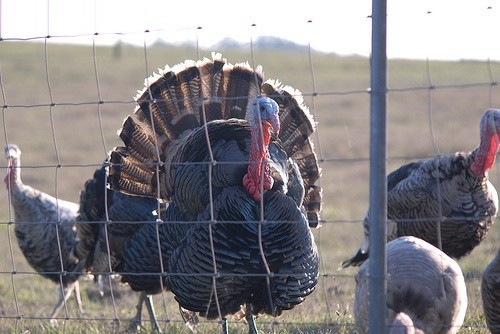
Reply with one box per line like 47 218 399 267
481 248 500 334
336 104 500 271
104 50 327 334
66 156 170 334
3 142 92 328
350 233 470 334
